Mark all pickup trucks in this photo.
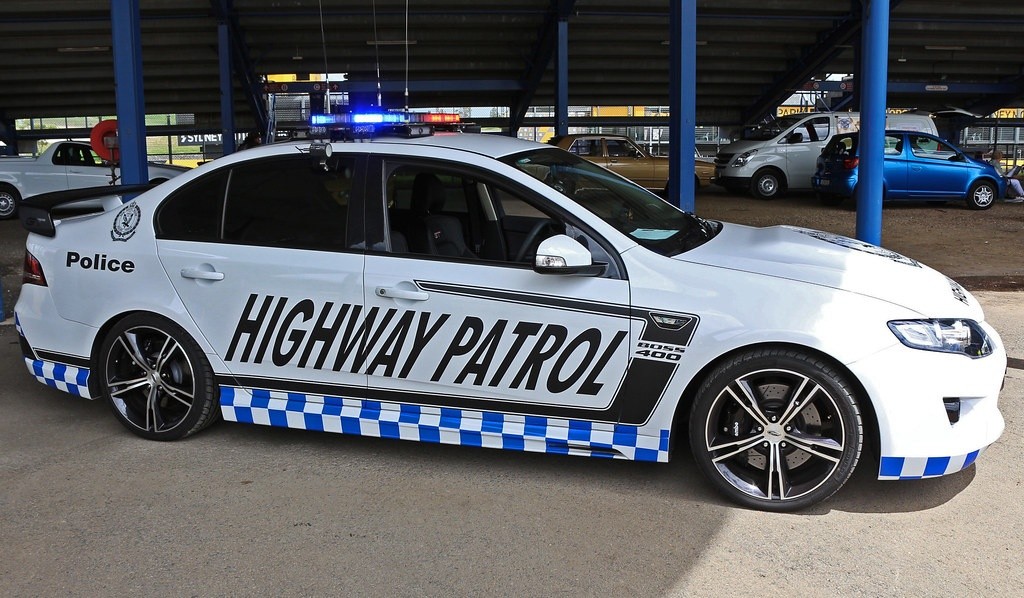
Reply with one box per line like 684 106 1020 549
0 139 194 225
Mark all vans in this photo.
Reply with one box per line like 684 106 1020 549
713 111 942 201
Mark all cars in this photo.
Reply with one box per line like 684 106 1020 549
810 130 1006 211
544 134 716 198
12 112 1008 515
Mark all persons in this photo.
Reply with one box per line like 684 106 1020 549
988 150 1024 197
238 132 263 155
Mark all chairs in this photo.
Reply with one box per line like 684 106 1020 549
61 147 71 165
387 229 407 253
410 174 478 258
71 147 81 166
